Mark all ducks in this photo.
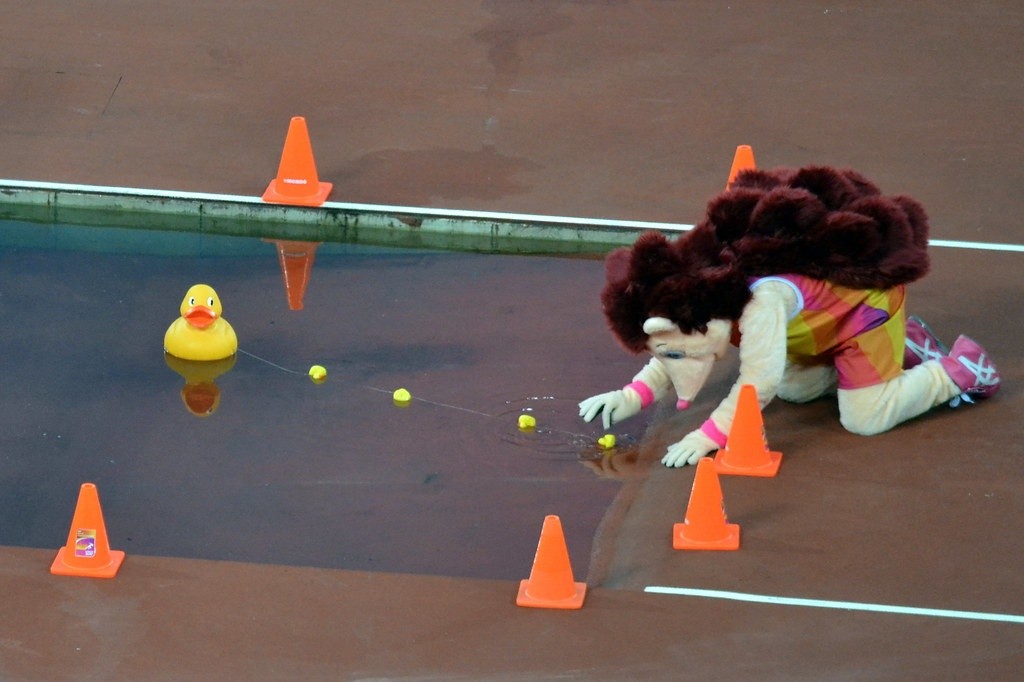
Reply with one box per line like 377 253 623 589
163 284 239 361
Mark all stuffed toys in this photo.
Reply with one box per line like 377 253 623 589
577 163 1001 468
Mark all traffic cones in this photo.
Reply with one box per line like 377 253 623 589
265 236 324 313
262 116 333 207
726 145 759 189
516 515 587 611
713 384 784 478
49 483 125 581
672 458 741 551
